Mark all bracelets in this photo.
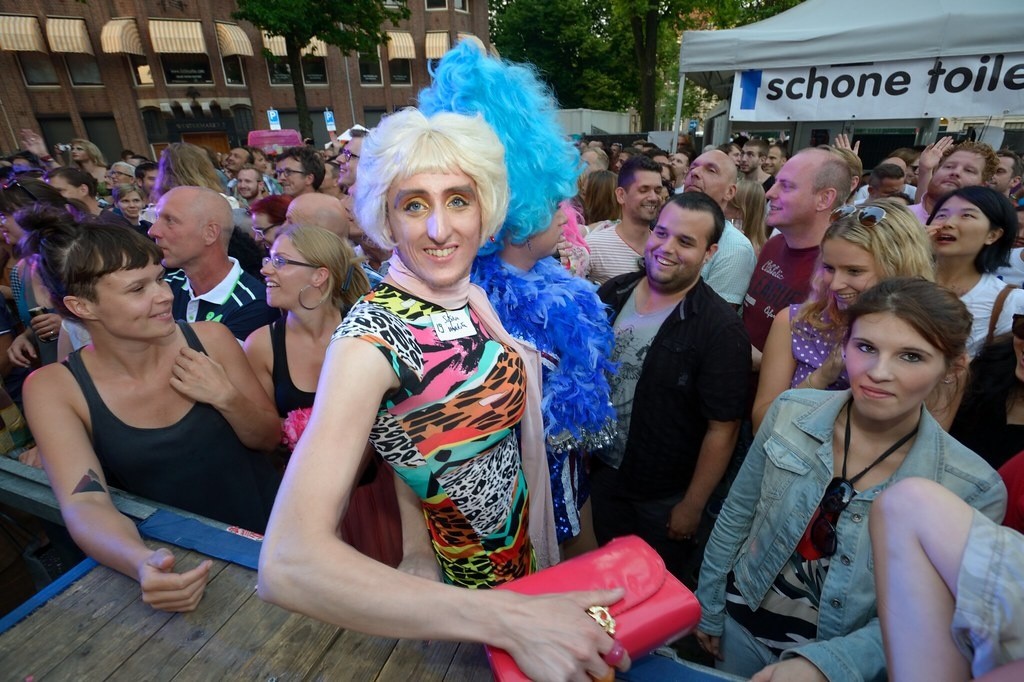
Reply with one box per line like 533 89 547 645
40 155 52 162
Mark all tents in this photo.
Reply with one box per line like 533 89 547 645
671 0 1024 153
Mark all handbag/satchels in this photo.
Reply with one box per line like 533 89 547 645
480 535 701 681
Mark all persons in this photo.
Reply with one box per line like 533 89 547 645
0 39 1024 682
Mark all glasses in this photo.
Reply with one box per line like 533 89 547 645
342 150 359 161
277 169 303 177
261 257 314 269
111 171 132 177
829 205 887 228
251 224 276 237
3 178 42 204
810 477 854 555
909 166 918 171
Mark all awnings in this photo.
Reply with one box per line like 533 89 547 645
0 14 485 61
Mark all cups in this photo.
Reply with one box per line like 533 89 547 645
29 307 56 342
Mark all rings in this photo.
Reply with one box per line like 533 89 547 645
604 639 624 666
594 666 615 682
50 330 54 336
684 536 690 539
30 138 35 141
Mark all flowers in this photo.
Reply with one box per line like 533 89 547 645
282 407 313 450
